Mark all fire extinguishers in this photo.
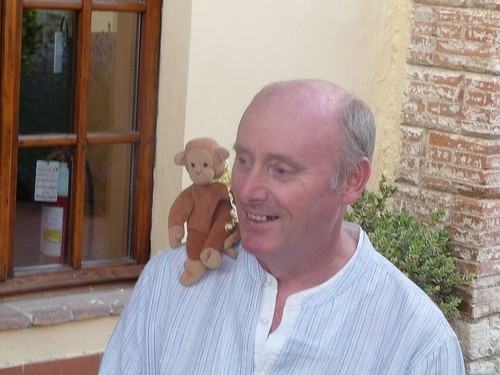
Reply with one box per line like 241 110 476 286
42 144 94 263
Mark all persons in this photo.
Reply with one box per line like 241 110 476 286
97 79 466 374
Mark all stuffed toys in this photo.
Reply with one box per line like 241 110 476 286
168 138 240 286
